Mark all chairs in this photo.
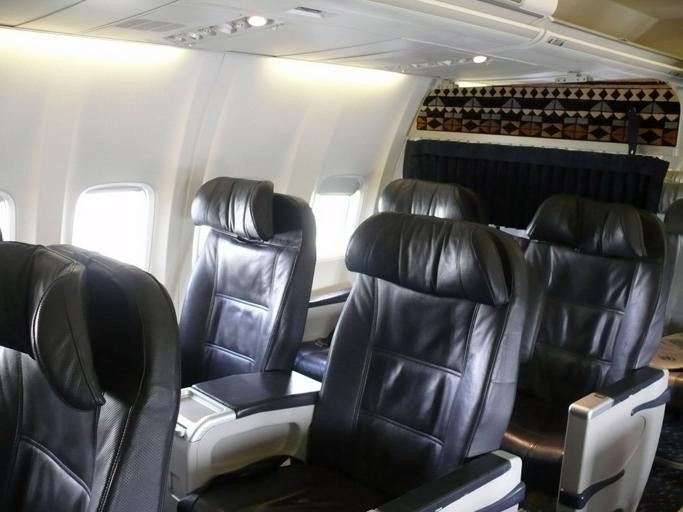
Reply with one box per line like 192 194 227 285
184 210 523 512
0 245 178 511
179 177 315 382
296 178 452 377
504 194 672 494
651 198 683 406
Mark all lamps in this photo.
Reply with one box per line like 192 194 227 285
473 56 487 64
247 16 266 28
